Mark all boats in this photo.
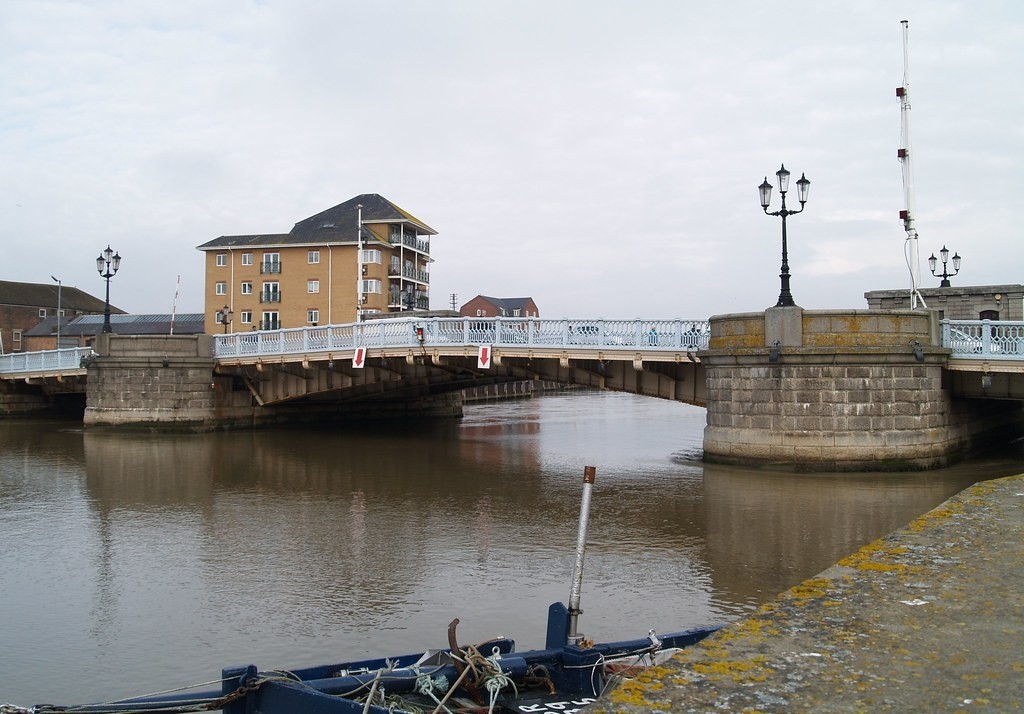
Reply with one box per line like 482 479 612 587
0 617 731 714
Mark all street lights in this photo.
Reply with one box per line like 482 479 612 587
758 161 811 308
96 244 122 332
50 274 62 349
929 244 962 287
353 203 363 321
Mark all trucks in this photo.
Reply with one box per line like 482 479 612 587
364 318 450 346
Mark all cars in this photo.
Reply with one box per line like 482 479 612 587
561 326 632 346
950 328 1002 355
458 325 530 345
